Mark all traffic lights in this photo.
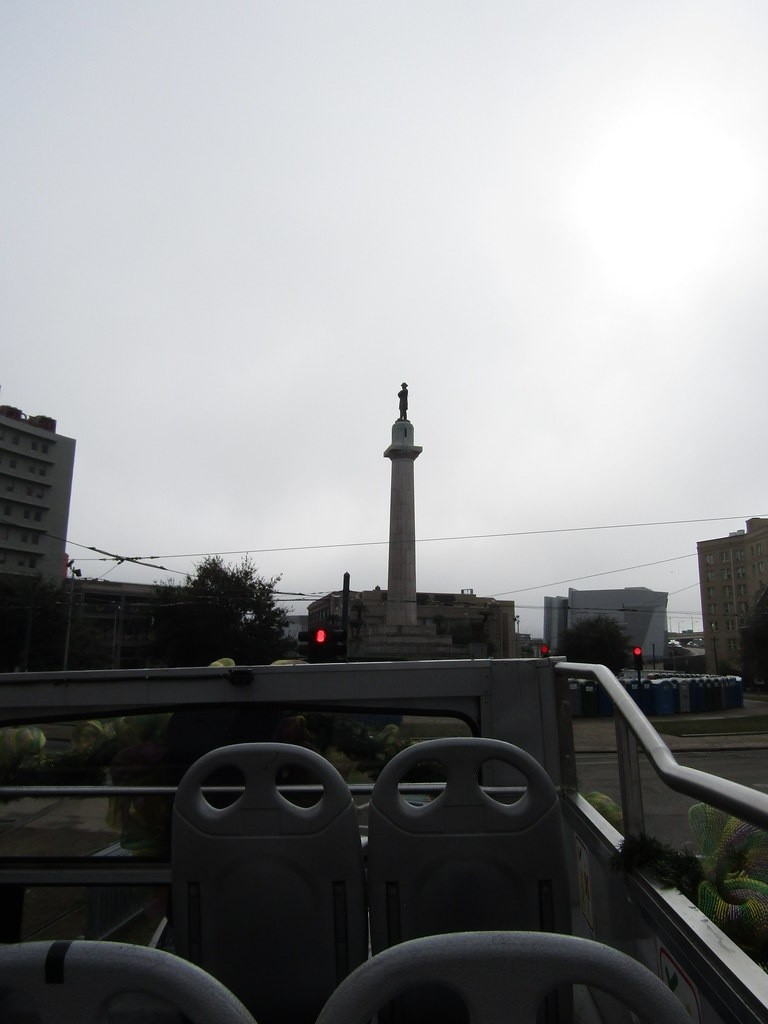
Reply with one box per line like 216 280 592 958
330 625 347 662
541 643 549 658
633 647 644 671
314 626 330 662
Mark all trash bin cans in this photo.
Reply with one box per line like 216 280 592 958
567 672 744 717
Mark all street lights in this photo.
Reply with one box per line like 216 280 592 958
62 559 82 670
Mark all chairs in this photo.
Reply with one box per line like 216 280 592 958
2 734 697 1023
